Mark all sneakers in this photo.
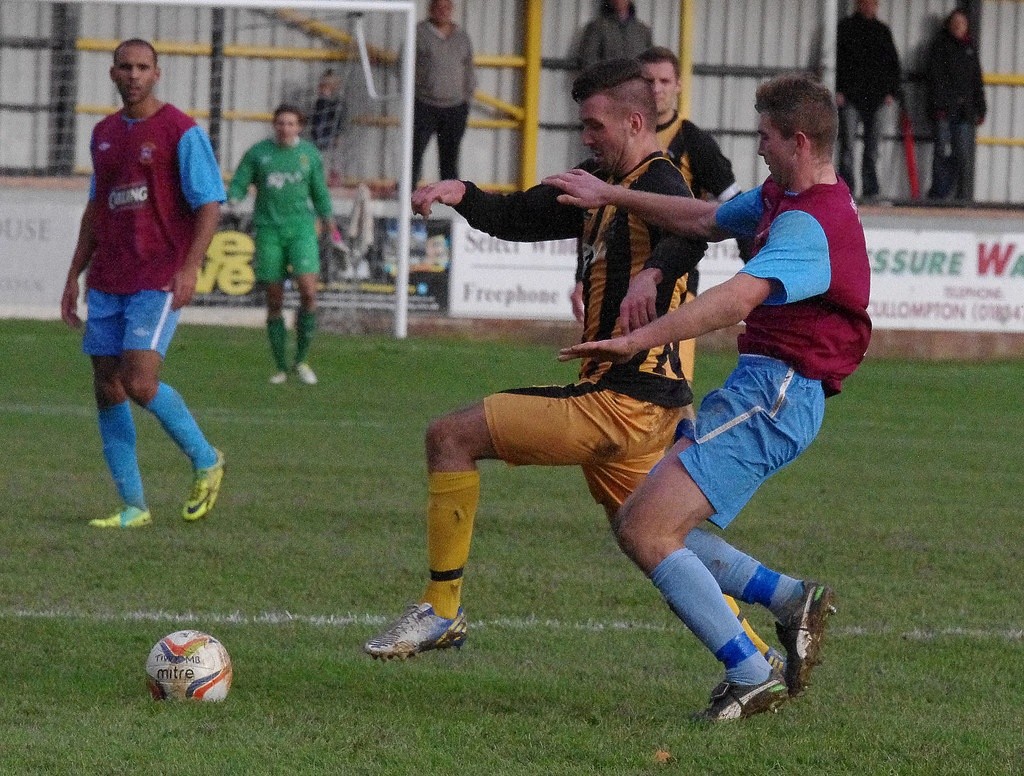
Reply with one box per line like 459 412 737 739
180 446 227 522
294 361 318 385
763 646 790 681
775 580 838 699
692 667 790 725
363 603 469 663
267 370 289 385
87 503 154 531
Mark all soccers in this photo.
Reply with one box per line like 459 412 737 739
145 629 233 704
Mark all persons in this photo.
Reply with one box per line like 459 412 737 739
570 0 740 420
61 38 228 526
833 0 902 206
395 0 476 195
311 67 348 191
227 104 350 384
543 81 874 720
916 8 986 206
363 57 788 673
